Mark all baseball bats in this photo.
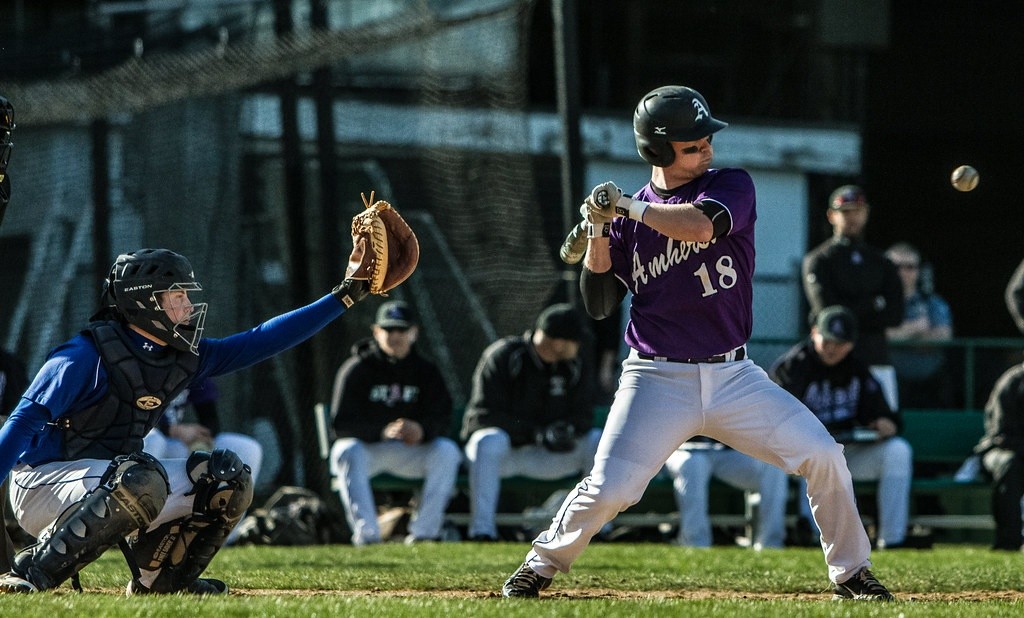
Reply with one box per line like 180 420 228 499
559 189 613 264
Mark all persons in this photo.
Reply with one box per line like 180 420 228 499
765 303 933 551
969 258 1024 553
503 86 895 605
456 303 616 546
1 190 421 605
882 242 954 344
137 377 265 547
665 440 788 550
798 185 897 411
328 301 461 546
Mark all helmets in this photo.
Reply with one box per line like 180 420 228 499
633 87 728 168
108 249 207 355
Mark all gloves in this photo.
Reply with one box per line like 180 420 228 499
580 181 651 239
536 421 575 454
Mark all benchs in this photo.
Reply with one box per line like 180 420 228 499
310 394 993 543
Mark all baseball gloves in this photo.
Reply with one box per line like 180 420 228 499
351 200 420 294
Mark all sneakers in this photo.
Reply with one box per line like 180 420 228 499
125 577 228 596
0 573 38 595
830 567 893 602
503 562 552 600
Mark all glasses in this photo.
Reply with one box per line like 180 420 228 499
380 327 410 334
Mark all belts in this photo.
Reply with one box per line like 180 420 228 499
638 347 745 364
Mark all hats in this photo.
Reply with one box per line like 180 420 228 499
818 306 856 343
376 302 416 327
830 185 869 211
537 302 596 342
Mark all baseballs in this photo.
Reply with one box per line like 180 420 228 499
952 165 979 191
